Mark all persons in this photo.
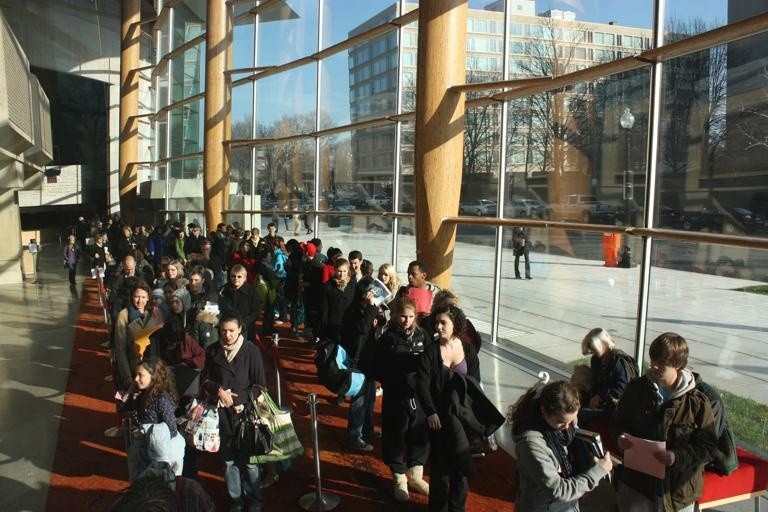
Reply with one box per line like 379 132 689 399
416 306 481 511
375 295 430 502
341 261 442 451
270 204 279 232
504 380 613 512
76 215 373 348
64 234 80 285
424 290 482 354
611 331 715 512
688 367 726 445
574 326 638 410
512 225 532 279
292 205 301 236
301 206 313 234
113 282 266 510
571 365 612 413
282 205 289 230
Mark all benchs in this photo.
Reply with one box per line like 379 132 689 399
585 417 768 512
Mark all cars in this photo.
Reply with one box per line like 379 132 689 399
582 203 764 237
260 196 358 212
460 198 496 216
506 198 547 219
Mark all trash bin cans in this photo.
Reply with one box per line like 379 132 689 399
603 231 622 265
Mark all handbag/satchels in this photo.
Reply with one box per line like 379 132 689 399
234 409 303 464
704 421 738 474
176 399 220 453
314 342 365 399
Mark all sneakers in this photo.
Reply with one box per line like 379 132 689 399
346 431 382 452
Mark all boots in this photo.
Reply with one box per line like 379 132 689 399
392 466 429 502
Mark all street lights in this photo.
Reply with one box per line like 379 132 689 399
617 106 640 270
282 150 290 194
326 153 341 228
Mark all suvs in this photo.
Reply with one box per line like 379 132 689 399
398 192 415 211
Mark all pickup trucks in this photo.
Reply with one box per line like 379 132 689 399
363 194 392 207
542 193 612 224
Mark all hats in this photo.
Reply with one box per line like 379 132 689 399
151 288 165 301
169 288 191 327
299 242 316 256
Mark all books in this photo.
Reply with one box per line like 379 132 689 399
622 429 669 480
572 429 612 485
